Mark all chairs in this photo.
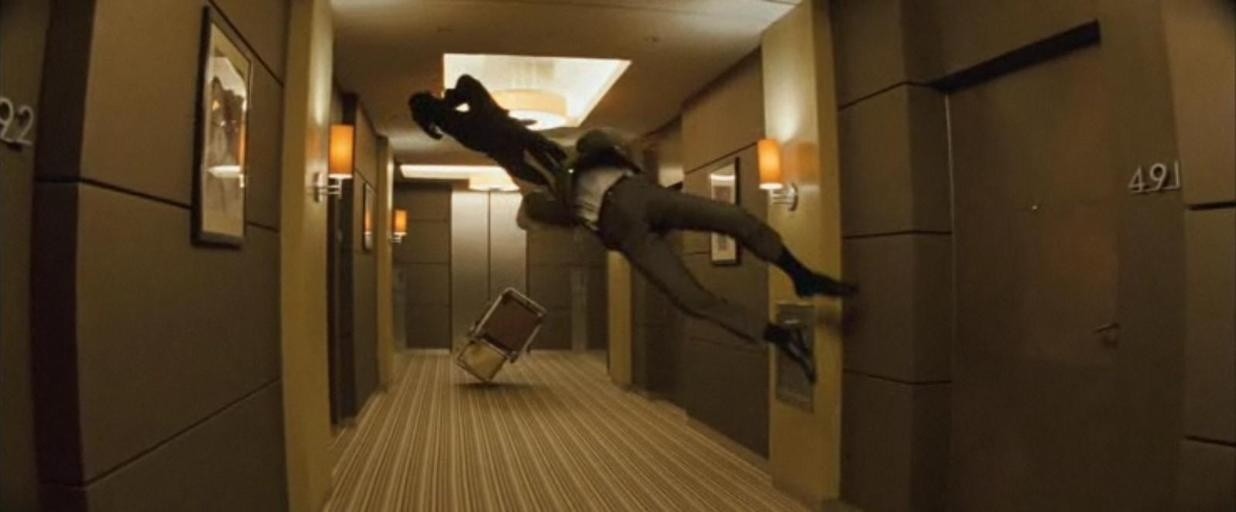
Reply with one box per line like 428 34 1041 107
453 286 550 385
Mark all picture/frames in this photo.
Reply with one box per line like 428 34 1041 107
706 157 742 266
362 180 379 251
188 7 255 248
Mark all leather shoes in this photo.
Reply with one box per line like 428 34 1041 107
779 328 814 380
796 277 855 297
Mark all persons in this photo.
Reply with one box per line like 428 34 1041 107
523 127 860 385
410 73 567 195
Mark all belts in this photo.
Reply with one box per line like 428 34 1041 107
598 174 627 229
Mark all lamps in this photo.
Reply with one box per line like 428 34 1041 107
389 208 408 245
312 123 354 201
756 138 798 211
492 89 567 132
